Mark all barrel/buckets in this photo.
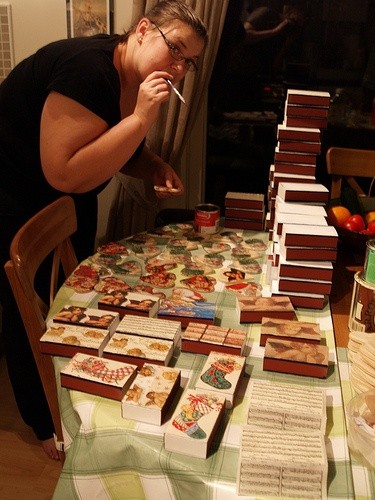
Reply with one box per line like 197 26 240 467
195 204 220 236
326 197 375 254
348 239 375 333
347 390 375 463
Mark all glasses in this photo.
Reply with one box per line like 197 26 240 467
151 21 199 73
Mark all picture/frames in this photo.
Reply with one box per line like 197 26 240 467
65 0 115 39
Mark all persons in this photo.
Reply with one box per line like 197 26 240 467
243 0 306 82
0 0 209 462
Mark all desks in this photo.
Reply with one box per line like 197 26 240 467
45 224 375 500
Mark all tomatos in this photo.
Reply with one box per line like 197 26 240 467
326 205 375 235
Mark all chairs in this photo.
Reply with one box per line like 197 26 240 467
326 148 375 199
4 195 81 434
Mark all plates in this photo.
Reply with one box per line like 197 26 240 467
347 331 375 393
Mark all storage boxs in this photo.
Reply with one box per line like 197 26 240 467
41 89 339 458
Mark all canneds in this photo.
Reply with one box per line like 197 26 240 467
194 202 221 234
358 239 375 287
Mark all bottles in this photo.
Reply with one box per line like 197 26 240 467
330 89 346 125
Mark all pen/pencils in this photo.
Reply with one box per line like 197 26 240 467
166 79 186 104
